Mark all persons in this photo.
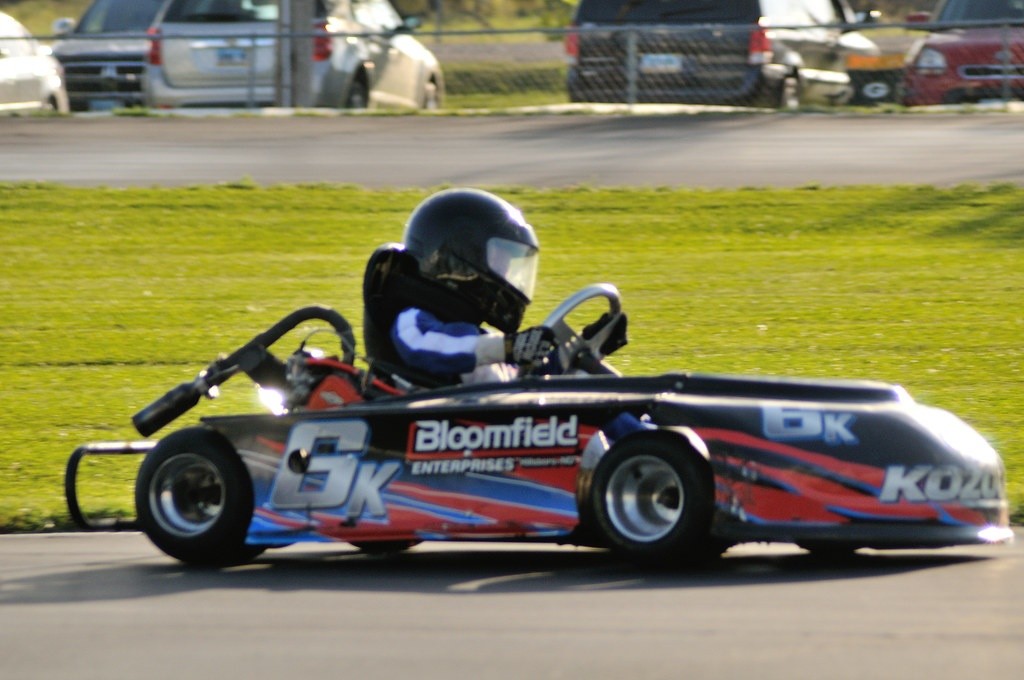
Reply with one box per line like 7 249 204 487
381 188 629 390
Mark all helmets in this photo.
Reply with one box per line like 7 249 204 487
402 188 539 336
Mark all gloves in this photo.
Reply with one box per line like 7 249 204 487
582 312 629 355
504 328 556 371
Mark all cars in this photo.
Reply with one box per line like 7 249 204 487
0 11 69 115
51 0 167 112
896 1 1024 108
564 0 879 108
142 0 445 110
